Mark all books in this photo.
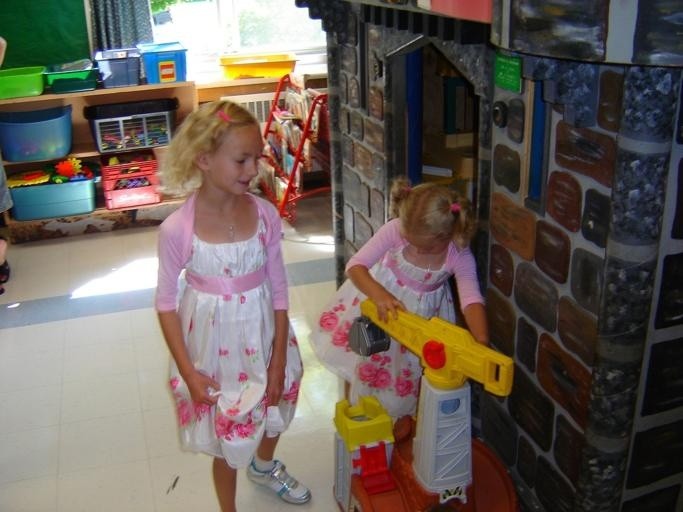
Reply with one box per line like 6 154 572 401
258 88 317 211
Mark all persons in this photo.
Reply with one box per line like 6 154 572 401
0 37 15 285
308 168 493 440
157 98 313 512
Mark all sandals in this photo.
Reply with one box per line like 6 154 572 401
0 260 9 283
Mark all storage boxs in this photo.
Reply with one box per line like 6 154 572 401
135 41 188 85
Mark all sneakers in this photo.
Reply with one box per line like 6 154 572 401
246 453 311 504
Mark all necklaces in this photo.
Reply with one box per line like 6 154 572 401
206 188 247 243
413 244 448 272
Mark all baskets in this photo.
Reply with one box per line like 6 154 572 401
100 149 162 210
84 99 179 154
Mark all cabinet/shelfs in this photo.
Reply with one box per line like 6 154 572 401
0 77 199 225
258 74 332 224
